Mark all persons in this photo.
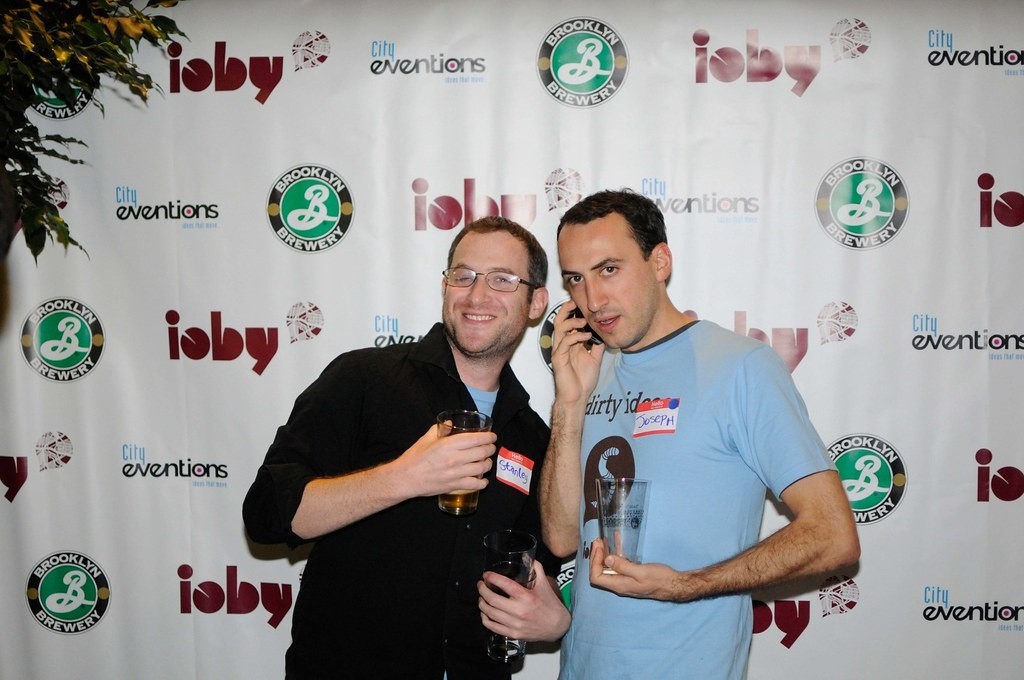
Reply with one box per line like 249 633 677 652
539 188 862 680
243 216 571 680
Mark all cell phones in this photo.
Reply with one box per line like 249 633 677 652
575 308 603 344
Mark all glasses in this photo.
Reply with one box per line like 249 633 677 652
442 265 537 292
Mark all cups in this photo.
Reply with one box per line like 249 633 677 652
483 528 538 661
595 477 651 574
438 409 494 516
